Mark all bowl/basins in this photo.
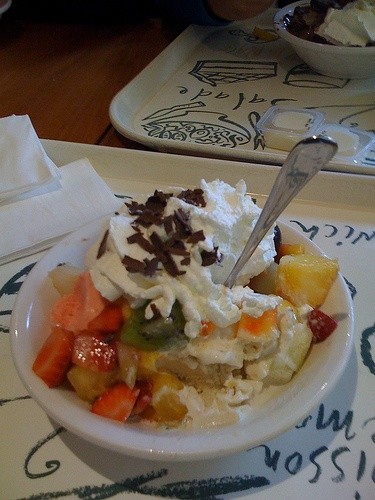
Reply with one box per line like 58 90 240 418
9 211 354 462
273 0 375 79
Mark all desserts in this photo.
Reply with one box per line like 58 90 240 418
32 179 340 434
